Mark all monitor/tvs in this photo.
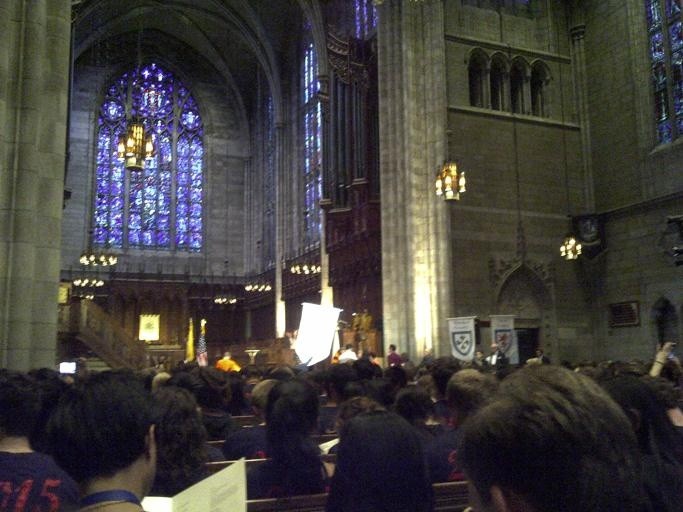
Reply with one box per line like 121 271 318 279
58 361 78 375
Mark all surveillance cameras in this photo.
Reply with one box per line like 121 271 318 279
224 258 229 264
64 186 72 200
672 246 683 256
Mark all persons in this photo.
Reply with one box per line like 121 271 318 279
1 338 682 511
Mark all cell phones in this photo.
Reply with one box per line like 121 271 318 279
667 351 673 358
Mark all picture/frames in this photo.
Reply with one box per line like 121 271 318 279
608 301 641 328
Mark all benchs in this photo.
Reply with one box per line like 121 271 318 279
198 387 473 512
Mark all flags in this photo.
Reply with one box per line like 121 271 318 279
185 318 195 363
195 317 210 367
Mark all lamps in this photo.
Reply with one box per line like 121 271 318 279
116 0 155 171
79 10 118 267
559 1 583 260
434 2 470 202
